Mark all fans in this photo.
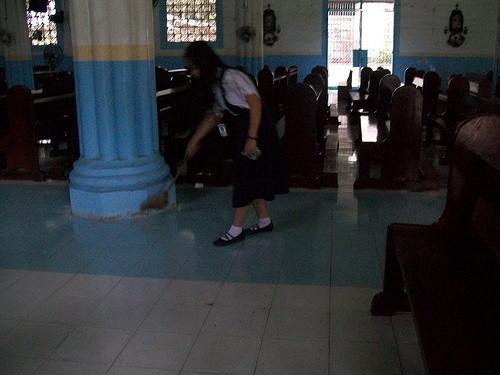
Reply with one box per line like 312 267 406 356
43 43 64 72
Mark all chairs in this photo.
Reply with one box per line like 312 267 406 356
371 114 499 375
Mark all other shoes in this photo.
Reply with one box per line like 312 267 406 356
247 219 274 234
213 229 245 245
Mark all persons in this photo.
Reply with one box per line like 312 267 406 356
220 127 225 135
183 41 291 247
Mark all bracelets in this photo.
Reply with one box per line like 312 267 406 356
246 135 257 140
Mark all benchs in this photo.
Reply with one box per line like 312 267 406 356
0 62 493 176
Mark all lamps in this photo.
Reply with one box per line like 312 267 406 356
263 4 276 46
446 4 465 47
